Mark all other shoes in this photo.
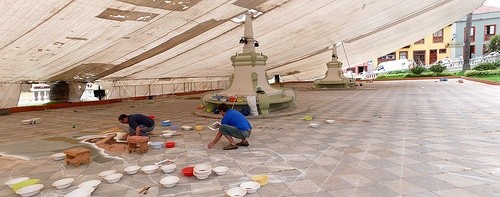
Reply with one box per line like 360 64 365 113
236 141 249 146
223 144 238 150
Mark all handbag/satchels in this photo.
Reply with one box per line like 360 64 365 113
247 95 258 115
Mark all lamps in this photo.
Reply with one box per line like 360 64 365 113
452 33 457 40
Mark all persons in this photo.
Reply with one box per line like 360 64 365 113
208 103 252 150
408 59 424 68
472 53 476 58
118 114 155 139
438 55 462 66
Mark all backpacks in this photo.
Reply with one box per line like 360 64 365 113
257 93 269 109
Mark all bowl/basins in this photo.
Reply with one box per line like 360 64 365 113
64 180 101 197
251 175 269 187
103 173 123 182
160 176 180 187
240 182 261 193
211 95 247 102
326 120 336 123
206 109 211 112
226 186 246 197
304 116 312 120
140 165 158 174
195 125 203 130
193 164 211 180
181 167 194 177
166 142 175 148
213 166 229 175
163 133 172 139
97 170 117 179
51 153 66 161
182 126 192 131
150 142 163 149
162 164 176 173
4 176 44 197
124 165 140 175
312 124 319 127
52 177 74 189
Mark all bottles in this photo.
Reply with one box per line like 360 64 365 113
214 106 219 114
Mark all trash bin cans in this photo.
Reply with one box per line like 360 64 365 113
275 75 279 82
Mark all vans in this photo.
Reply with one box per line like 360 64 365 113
376 59 414 71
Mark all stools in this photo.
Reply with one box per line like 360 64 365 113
127 135 150 153
64 148 90 167
